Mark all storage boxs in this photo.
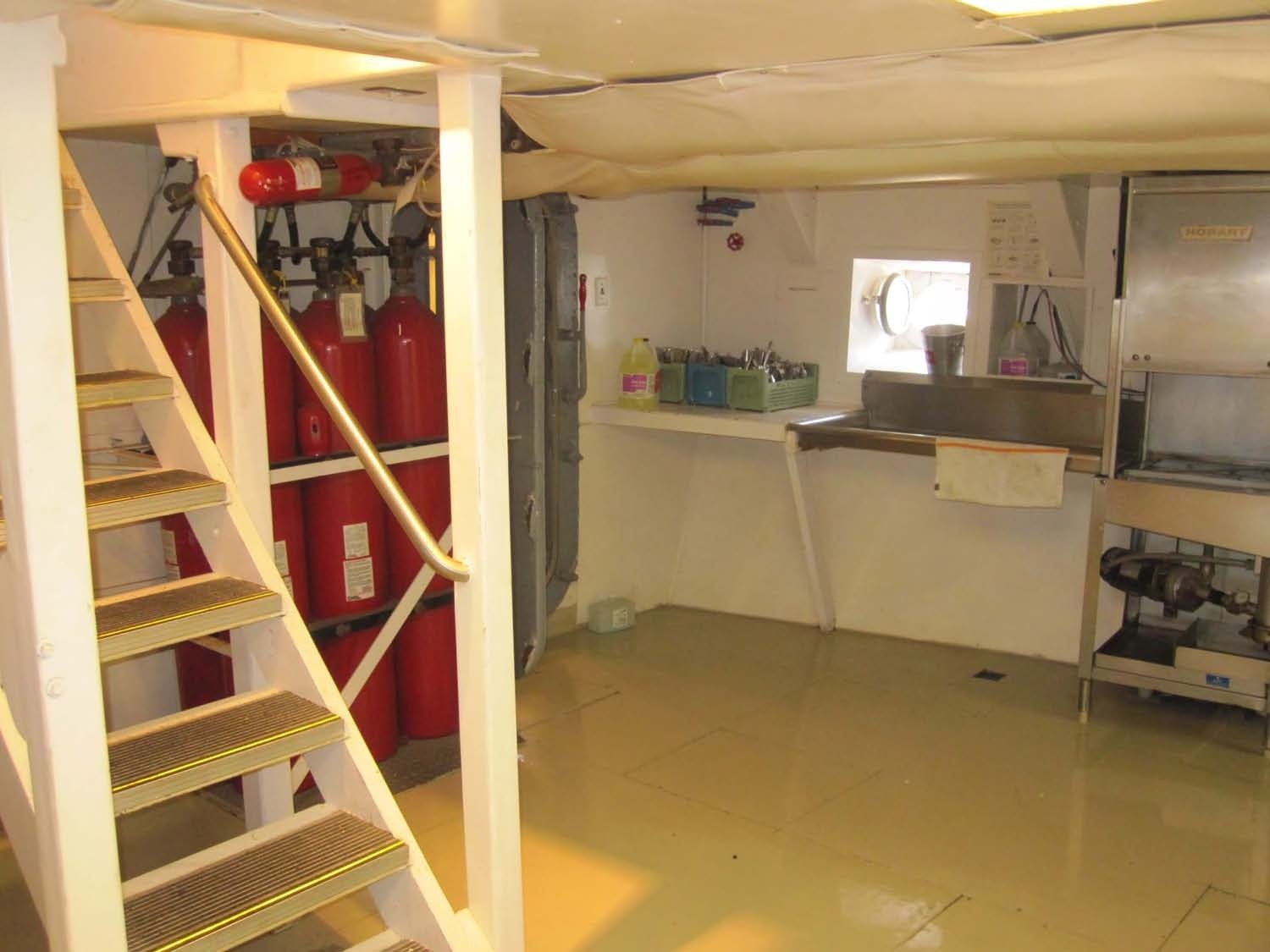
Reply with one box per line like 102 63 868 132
658 362 820 413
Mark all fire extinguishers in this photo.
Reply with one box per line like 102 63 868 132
239 152 388 207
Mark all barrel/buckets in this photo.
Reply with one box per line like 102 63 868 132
921 324 965 382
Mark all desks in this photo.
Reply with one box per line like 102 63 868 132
589 402 852 634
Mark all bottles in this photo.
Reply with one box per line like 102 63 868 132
618 336 659 413
